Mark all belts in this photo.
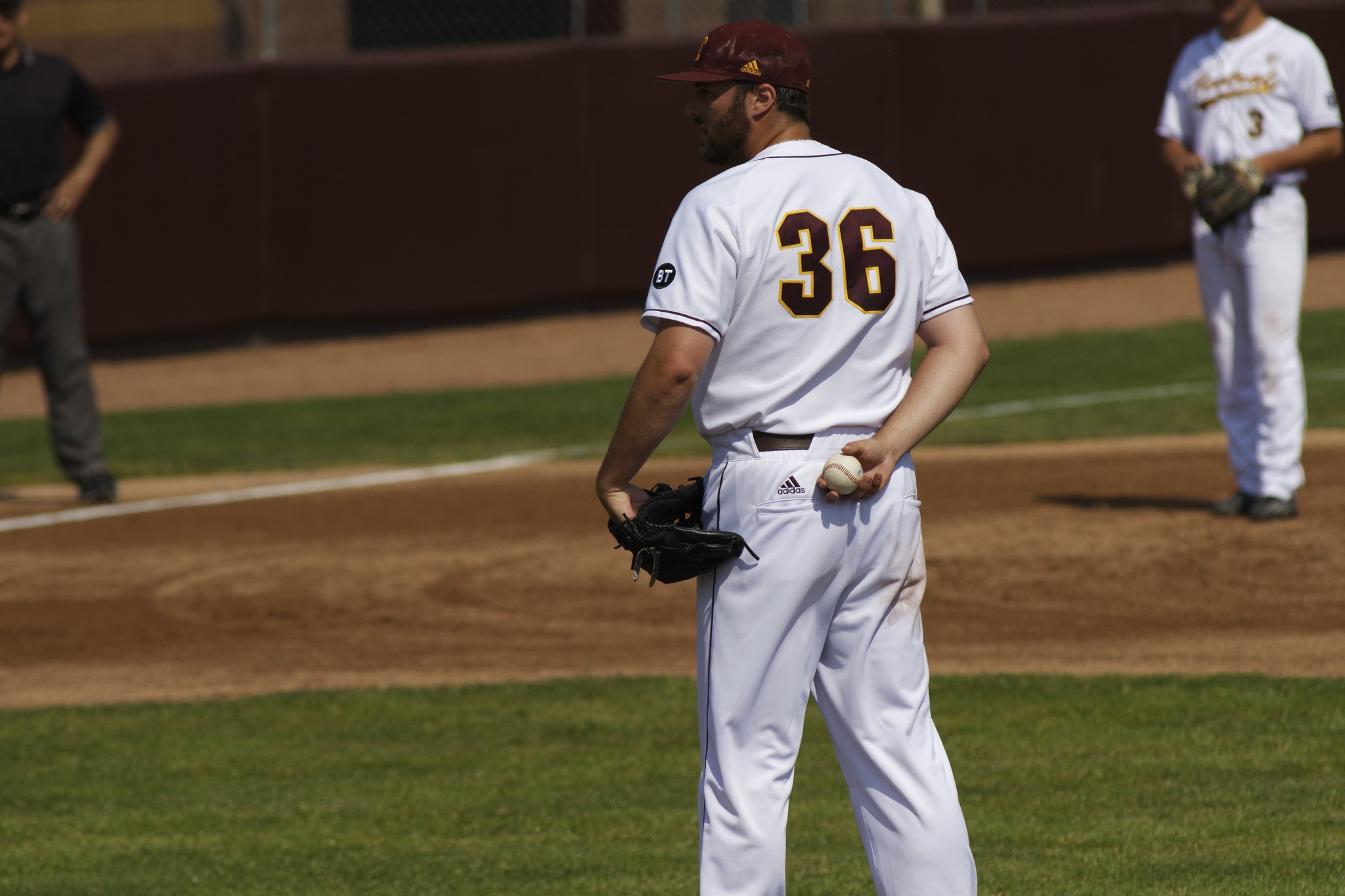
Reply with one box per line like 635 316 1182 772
751 429 812 452
1258 182 1272 196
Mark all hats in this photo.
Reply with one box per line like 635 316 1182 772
655 19 812 91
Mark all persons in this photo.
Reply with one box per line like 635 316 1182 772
1157 0 1345 523
594 22 992 896
0 1 123 505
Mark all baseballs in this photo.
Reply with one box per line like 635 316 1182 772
822 452 864 494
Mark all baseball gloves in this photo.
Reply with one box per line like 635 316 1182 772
606 479 744 584
1183 157 1267 233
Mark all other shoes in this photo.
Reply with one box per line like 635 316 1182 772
77 472 115 504
1250 498 1294 518
1213 493 1250 515
